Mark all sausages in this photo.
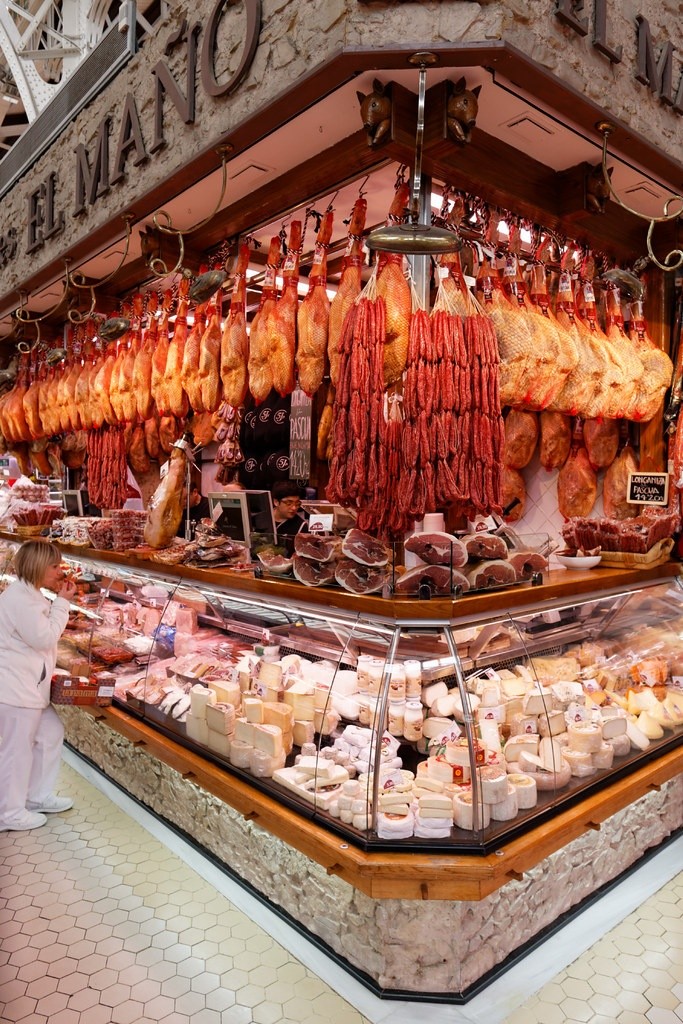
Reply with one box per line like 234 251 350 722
12 507 63 526
84 428 129 509
323 294 504 531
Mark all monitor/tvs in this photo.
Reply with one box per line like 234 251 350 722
62 490 84 518
208 490 278 565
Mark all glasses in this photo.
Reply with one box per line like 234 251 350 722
276 498 302 509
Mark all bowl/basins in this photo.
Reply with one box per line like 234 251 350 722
556 554 603 571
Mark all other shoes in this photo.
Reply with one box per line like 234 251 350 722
25 795 74 813
0 808 48 832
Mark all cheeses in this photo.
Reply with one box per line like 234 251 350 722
127 605 683 841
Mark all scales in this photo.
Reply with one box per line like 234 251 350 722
0 452 65 512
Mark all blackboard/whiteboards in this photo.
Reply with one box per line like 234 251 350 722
627 470 668 505
289 390 312 480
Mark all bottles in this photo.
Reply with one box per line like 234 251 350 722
356 654 424 743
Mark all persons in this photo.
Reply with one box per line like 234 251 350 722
177 483 210 542
1 541 77 832
272 482 309 534
